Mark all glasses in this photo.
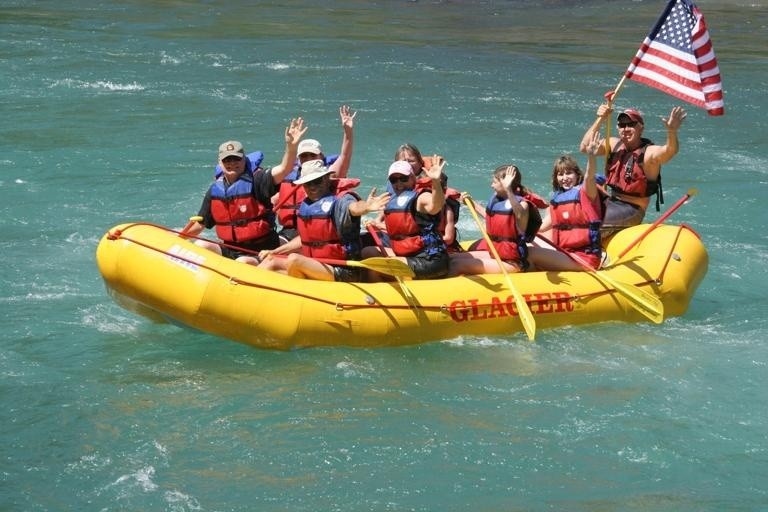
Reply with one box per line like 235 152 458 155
389 175 410 184
618 121 638 129
305 175 324 186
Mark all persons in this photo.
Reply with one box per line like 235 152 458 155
271 106 357 247
526 131 606 271
256 160 391 282
435 172 462 254
362 142 447 246
445 165 542 279
361 154 449 282
579 105 687 228
177 117 308 266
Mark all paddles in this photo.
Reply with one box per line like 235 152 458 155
367 223 419 316
274 254 415 278
460 191 536 342
538 233 664 324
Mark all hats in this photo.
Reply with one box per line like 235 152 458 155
296 138 323 159
616 108 644 126
292 159 337 186
218 140 245 162
426 172 447 188
387 161 415 179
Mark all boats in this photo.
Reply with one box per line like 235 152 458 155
96 216 709 353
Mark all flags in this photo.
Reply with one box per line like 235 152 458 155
623 0 725 116
